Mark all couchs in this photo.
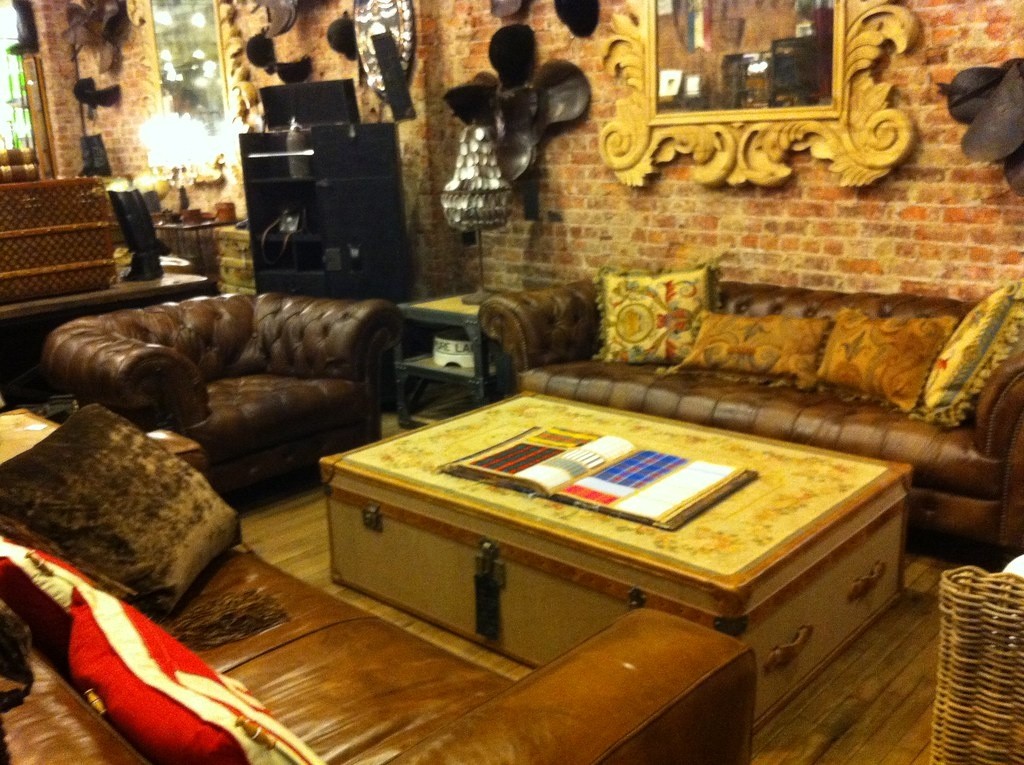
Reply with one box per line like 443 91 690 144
43 292 403 511
2 428 756 765
478 277 1024 572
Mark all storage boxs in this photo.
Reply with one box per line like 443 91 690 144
0 178 118 302
213 226 256 296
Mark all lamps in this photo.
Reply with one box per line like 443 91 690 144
440 118 514 304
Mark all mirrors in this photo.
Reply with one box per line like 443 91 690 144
598 0 920 187
135 1 262 135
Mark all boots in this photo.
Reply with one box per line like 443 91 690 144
107 188 163 283
79 134 111 177
7 0 39 55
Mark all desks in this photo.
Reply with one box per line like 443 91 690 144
0 273 208 320
155 220 238 276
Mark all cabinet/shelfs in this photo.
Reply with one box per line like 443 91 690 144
722 39 819 108
240 123 418 410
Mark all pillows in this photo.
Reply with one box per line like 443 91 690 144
593 267 725 366
67 585 330 765
816 307 958 414
656 309 831 393
2 540 104 667
0 403 241 619
907 281 1023 429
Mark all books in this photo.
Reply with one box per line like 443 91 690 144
438 426 758 532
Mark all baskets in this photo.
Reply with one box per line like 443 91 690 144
932 564 1024 765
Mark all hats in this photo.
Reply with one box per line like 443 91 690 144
84 85 120 107
246 35 275 76
489 23 534 90
328 19 356 61
275 56 313 85
554 0 600 39
73 78 97 109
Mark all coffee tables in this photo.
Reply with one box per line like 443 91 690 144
321 391 912 729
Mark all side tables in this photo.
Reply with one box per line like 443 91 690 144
0 408 63 466
394 294 497 428
